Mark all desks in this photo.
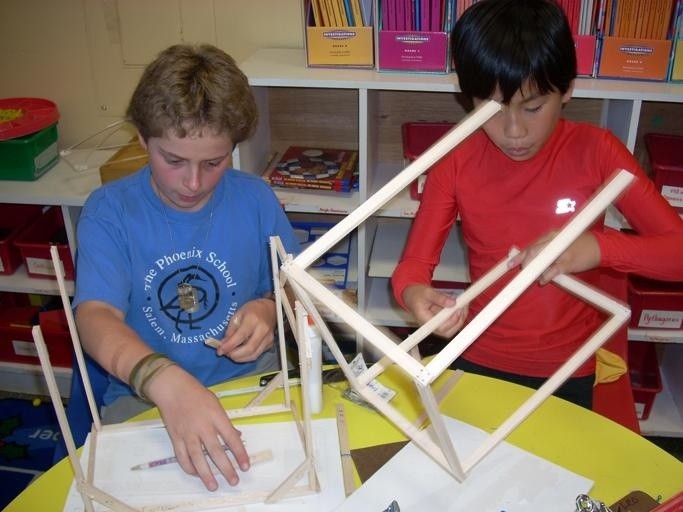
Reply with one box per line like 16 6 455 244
0 360 683 509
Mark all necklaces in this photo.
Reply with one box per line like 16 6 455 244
154 183 218 315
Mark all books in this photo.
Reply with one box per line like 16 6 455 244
302 1 682 39
255 144 358 195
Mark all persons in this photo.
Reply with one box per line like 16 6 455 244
388 0 682 415
53 44 304 490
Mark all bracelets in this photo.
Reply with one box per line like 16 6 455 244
127 352 177 404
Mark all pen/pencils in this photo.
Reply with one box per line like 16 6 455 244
131 441 245 470
